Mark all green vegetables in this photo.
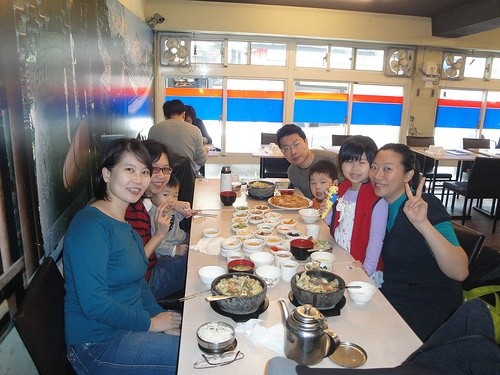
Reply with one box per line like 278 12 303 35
232 222 247 228
237 206 248 210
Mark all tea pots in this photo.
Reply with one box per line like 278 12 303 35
279 300 340 365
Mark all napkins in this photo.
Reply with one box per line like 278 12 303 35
189 236 224 255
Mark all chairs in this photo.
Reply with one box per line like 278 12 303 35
170 156 195 231
12 257 78 375
332 135 349 145
260 157 291 178
451 222 500 307
407 136 452 206
441 157 500 234
262 132 279 145
457 138 491 199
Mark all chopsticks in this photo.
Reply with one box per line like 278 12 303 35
178 289 212 301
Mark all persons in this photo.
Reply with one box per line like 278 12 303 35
62 137 191 375
277 124 469 344
184 104 212 178
148 99 208 176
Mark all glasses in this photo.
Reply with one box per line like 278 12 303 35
282 141 300 153
151 167 173 174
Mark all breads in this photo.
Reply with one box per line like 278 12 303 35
271 194 309 208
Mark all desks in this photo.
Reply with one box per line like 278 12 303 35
175 178 425 375
467 147 500 218
205 145 340 165
410 146 489 220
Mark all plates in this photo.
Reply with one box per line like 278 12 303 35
197 339 237 355
210 297 269 322
288 290 346 313
268 195 313 211
246 192 274 200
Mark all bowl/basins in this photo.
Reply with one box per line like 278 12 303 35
220 191 237 205
195 320 236 350
247 180 275 197
291 271 345 309
210 273 268 315
198 205 338 289
348 281 377 304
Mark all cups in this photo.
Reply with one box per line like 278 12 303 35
220 166 232 191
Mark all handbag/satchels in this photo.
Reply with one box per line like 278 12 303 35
462 284 500 345
461 169 471 182
